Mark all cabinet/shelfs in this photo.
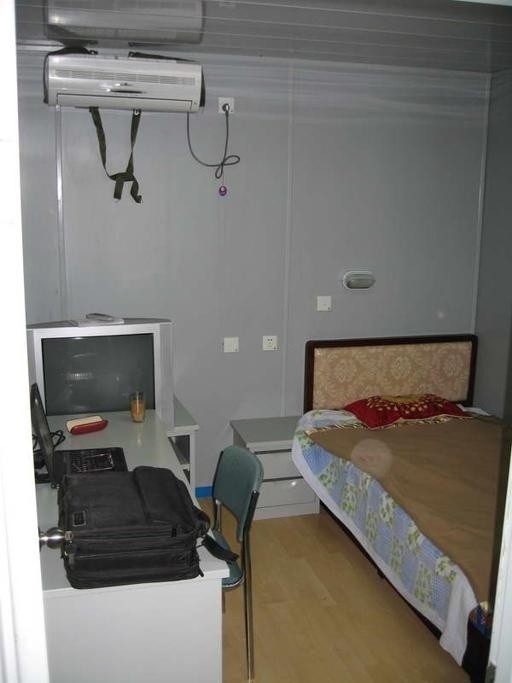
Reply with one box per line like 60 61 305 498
167 392 200 496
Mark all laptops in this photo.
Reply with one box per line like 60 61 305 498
30 382 128 483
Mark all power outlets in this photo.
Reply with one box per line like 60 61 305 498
263 335 278 350
217 95 234 112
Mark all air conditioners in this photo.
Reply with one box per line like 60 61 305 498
44 53 203 112
44 0 205 45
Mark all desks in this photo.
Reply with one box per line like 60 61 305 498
30 409 231 682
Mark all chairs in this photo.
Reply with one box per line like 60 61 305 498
203 444 266 683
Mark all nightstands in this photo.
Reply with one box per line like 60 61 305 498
227 414 321 519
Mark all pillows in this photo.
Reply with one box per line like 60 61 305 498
341 391 476 430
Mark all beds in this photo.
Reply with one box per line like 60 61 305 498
289 332 507 683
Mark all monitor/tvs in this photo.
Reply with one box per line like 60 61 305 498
26 319 175 430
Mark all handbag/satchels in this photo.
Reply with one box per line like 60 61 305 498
55 464 240 592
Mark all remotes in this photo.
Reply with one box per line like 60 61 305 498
85 313 115 321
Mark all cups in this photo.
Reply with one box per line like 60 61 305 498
129 391 147 422
